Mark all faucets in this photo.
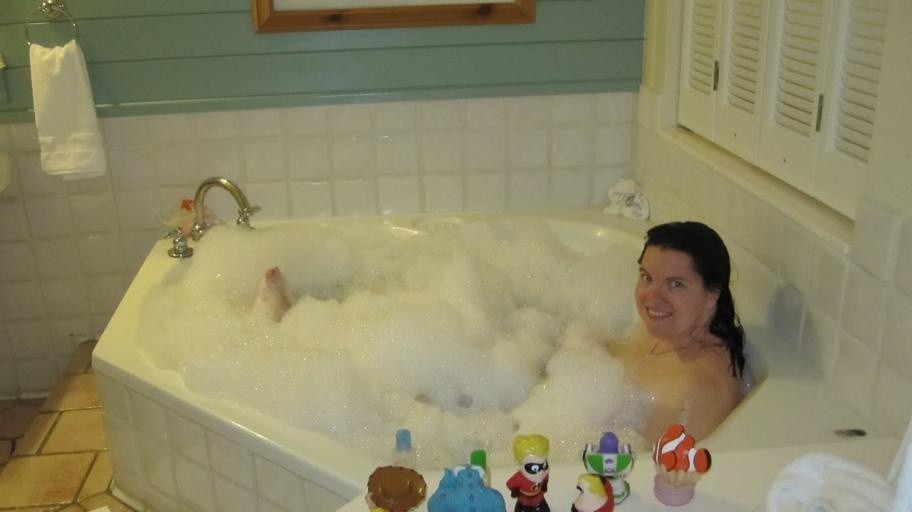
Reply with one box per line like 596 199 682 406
188 174 262 243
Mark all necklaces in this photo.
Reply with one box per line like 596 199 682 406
646 338 694 357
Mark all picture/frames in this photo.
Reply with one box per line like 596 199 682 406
250 1 538 33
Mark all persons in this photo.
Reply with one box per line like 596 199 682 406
257 215 751 455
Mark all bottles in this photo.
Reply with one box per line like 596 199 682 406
462 447 494 491
387 428 418 471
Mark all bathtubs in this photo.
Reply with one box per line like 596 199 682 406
95 206 824 512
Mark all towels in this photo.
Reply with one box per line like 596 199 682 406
30 40 110 180
604 181 647 222
